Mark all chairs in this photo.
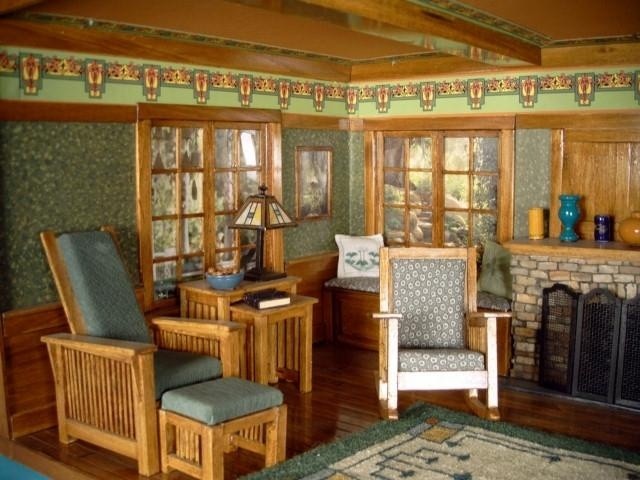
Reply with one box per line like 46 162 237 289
373 245 502 423
39 225 241 477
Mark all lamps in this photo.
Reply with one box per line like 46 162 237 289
227 183 298 282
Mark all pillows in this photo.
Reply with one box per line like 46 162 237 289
334 233 386 280
477 241 512 301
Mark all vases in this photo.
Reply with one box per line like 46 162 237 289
558 194 582 242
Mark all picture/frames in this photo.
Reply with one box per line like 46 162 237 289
294 145 334 223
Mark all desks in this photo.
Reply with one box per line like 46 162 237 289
177 270 303 321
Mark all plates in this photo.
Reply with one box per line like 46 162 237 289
206 268 243 289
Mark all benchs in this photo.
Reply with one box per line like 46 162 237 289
322 284 513 378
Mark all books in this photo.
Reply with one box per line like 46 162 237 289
244 288 291 311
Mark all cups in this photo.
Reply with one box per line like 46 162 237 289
528 208 544 240
595 214 610 242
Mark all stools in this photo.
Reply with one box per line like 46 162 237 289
158 374 288 480
230 294 320 394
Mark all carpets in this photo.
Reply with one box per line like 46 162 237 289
236 402 639 480
